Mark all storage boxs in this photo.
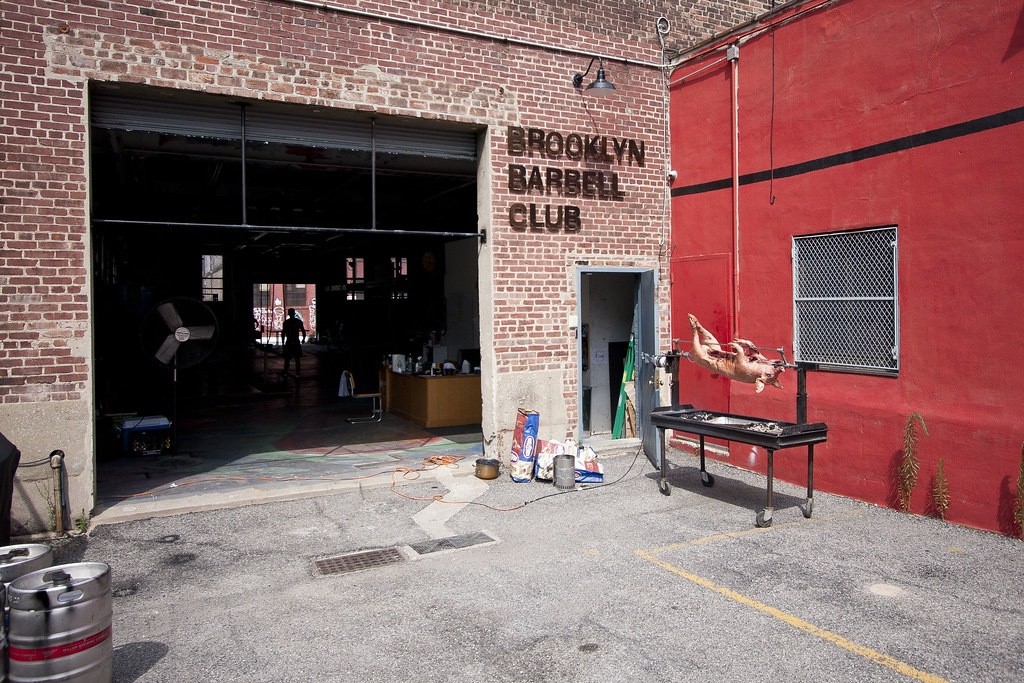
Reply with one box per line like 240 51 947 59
114 414 173 454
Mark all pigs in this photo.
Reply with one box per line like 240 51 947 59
687 312 785 393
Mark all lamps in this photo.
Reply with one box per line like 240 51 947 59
573 56 618 97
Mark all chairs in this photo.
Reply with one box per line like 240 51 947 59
345 372 384 424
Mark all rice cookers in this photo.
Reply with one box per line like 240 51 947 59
472 458 501 479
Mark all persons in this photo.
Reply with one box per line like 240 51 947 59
282 308 306 378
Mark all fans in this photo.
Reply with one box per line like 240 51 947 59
143 296 220 469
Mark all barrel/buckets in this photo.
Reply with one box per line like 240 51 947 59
0 543 112 683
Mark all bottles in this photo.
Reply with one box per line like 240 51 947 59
408 353 412 363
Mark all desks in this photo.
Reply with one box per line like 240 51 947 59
378 362 482 427
